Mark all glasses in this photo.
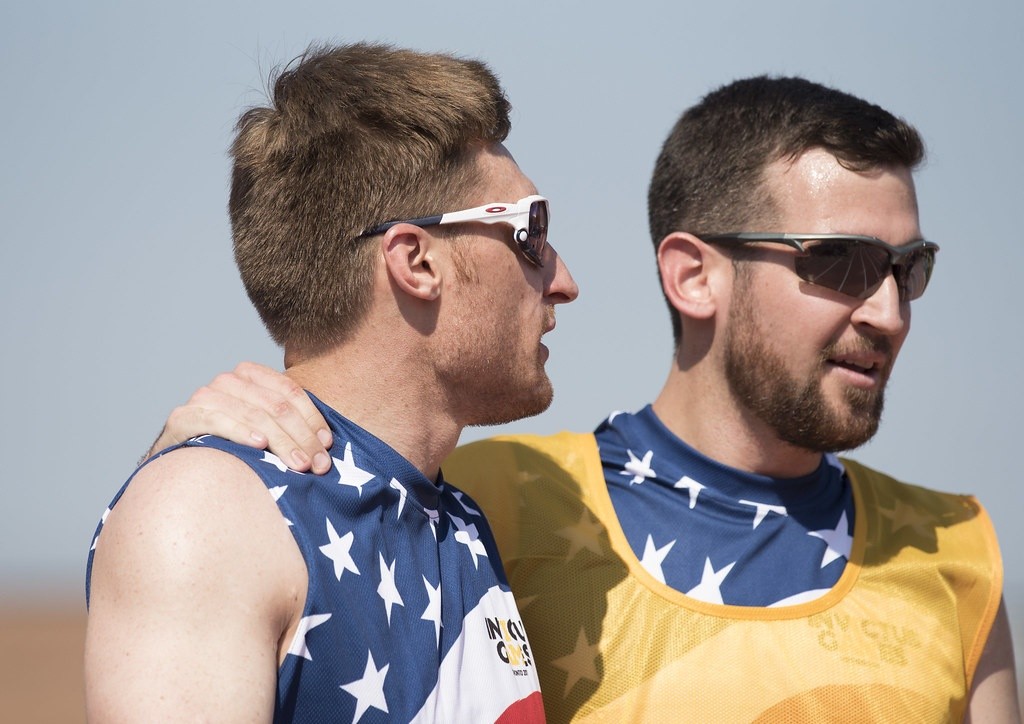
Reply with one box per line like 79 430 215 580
688 234 940 303
358 195 550 267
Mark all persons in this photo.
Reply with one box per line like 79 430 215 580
81 40 581 724
139 74 1023 724
